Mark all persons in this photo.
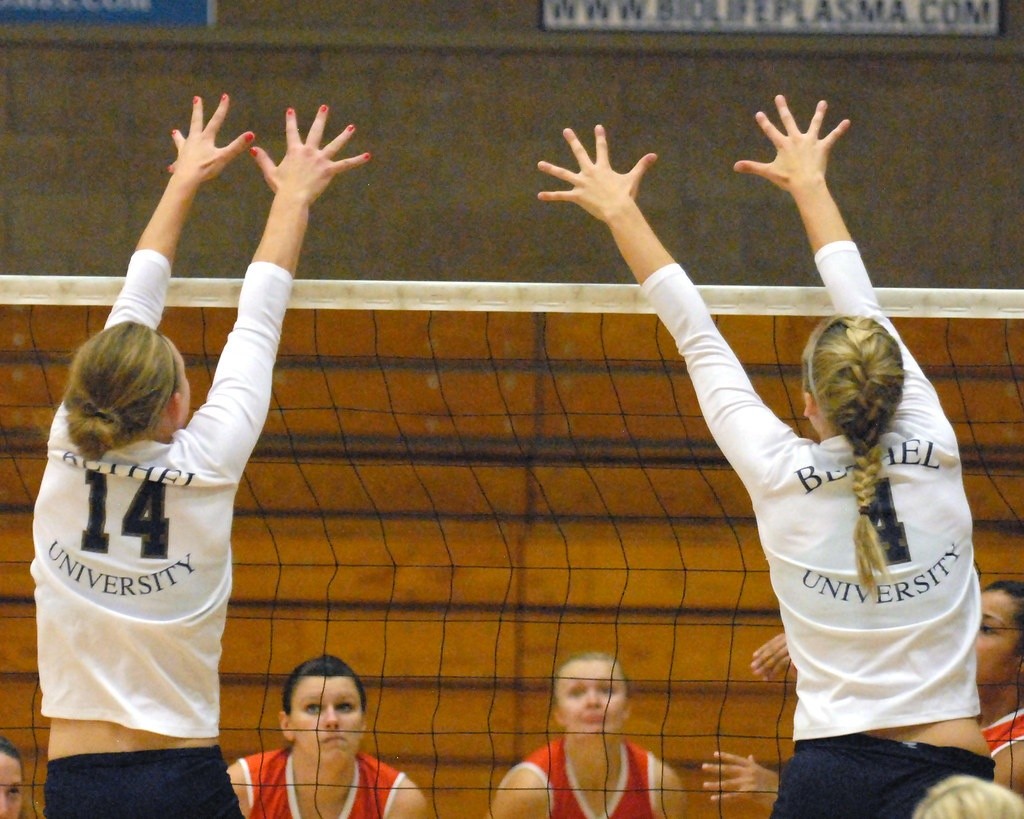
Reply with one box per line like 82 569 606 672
226 653 428 819
486 650 685 819
537 94 1024 819
0 93 371 819
